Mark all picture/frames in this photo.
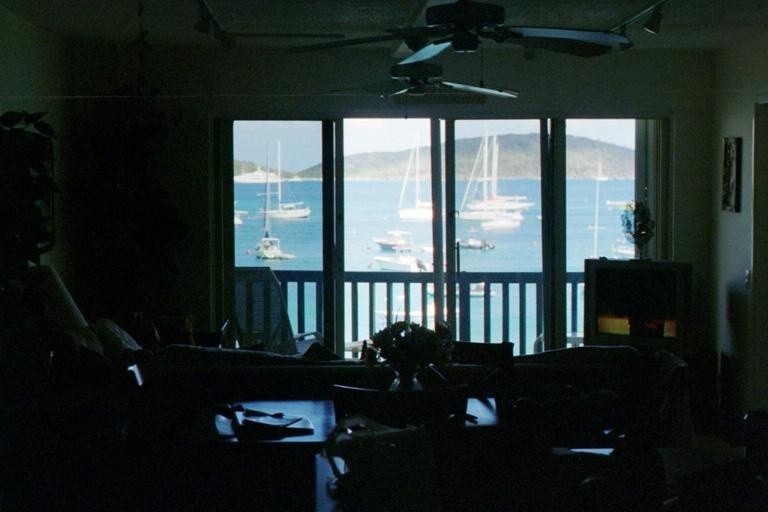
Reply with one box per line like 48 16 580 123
721 136 741 212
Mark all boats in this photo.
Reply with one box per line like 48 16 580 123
584 162 635 260
361 235 497 323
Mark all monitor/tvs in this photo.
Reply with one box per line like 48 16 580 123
583 259 695 357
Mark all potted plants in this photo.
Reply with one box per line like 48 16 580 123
621 202 656 269
370 321 448 398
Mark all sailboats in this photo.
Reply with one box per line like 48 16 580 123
458 129 538 229
257 142 310 219
393 137 434 222
254 148 281 259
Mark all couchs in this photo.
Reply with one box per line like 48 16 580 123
28 264 691 508
